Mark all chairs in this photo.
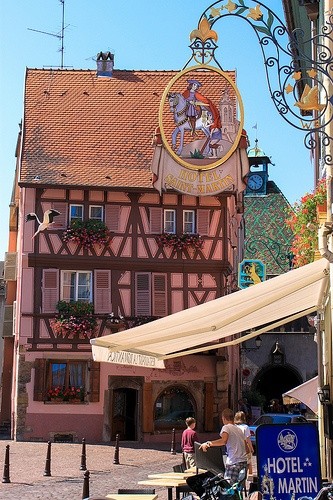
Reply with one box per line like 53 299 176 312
173 462 195 500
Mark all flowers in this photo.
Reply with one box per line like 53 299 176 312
66 385 83 402
159 232 203 252
61 218 111 249
48 385 65 402
51 301 97 337
282 178 327 269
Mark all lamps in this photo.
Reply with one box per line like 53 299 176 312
240 335 261 355
318 384 330 405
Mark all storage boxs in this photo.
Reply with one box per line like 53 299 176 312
54 433 73 443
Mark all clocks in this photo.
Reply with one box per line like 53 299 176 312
248 174 263 190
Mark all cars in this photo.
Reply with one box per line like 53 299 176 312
240 414 319 452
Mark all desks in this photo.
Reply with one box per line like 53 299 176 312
105 466 207 500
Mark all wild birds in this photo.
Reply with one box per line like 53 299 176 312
26 208 62 239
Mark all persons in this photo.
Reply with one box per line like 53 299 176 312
199 408 250 483
181 417 200 469
234 411 254 474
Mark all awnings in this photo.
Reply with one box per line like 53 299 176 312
89 257 330 369
282 374 318 415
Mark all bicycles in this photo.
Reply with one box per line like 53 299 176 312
181 473 262 500
